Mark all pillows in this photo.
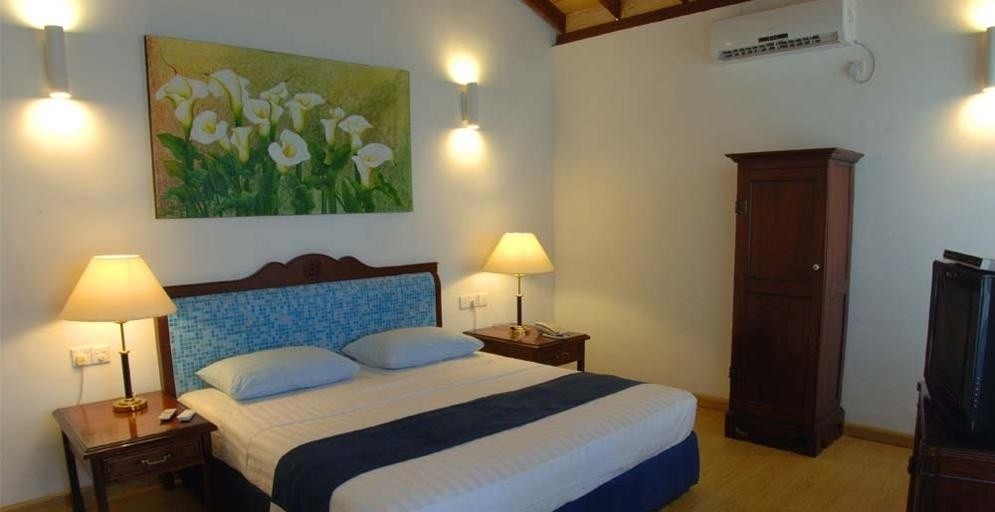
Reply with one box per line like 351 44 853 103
189 343 365 403
340 324 486 371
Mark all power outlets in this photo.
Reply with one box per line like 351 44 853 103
458 293 478 311
68 344 94 370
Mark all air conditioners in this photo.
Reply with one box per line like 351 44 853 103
705 0 860 65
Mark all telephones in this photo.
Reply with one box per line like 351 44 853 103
535 321 569 336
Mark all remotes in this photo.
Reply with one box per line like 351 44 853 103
158 408 177 421
176 409 197 421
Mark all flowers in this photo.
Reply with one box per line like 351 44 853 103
151 50 403 216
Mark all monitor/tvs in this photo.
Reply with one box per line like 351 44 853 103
923 257 994 447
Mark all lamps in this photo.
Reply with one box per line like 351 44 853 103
480 228 557 338
458 80 484 128
38 23 74 102
982 24 995 92
462 322 594 376
51 252 180 416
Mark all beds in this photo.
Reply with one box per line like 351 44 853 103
154 251 703 512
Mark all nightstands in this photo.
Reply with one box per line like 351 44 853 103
44 387 225 512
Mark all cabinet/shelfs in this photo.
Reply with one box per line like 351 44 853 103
717 146 870 460
899 380 995 510
